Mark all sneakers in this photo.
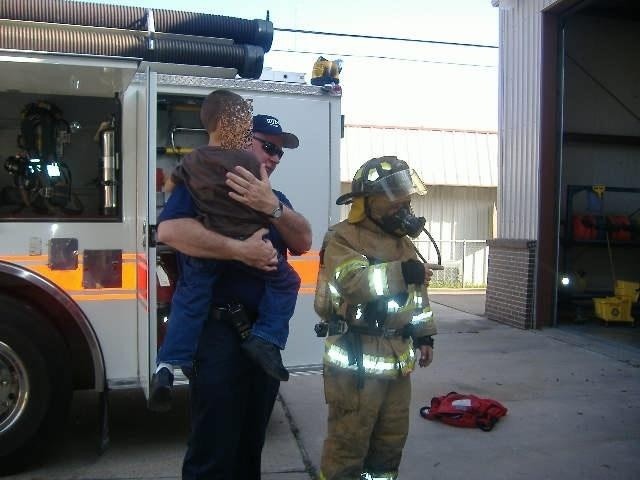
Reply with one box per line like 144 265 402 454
241 334 290 382
146 367 175 415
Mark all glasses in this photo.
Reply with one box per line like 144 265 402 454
251 136 284 160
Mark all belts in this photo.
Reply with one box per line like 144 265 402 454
348 324 403 338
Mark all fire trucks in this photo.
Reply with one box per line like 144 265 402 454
2 0 341 459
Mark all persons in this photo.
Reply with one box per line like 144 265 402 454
147 90 300 412
152 109 314 478
310 154 438 480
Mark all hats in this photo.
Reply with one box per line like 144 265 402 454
250 114 299 149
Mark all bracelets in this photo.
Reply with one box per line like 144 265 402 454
266 200 284 219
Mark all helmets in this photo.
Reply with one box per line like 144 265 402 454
352 155 415 225
